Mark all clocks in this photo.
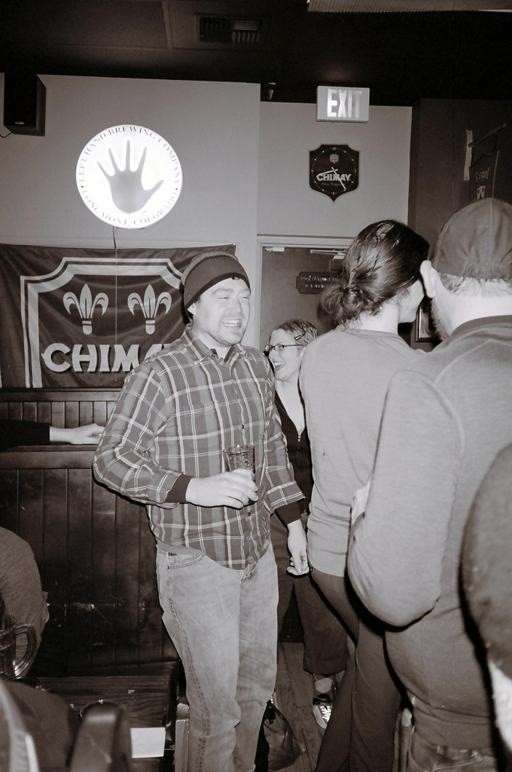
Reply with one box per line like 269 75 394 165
308 143 360 203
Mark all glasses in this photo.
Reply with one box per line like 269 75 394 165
264 344 304 352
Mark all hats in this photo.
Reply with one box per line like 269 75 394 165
432 198 512 279
181 250 250 319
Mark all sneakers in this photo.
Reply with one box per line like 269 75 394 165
313 694 332 729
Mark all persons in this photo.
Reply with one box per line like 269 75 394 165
0 419 105 452
260 310 352 739
337 191 512 772
88 249 311 772
295 217 434 772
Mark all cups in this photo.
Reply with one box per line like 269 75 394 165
223 445 257 507
